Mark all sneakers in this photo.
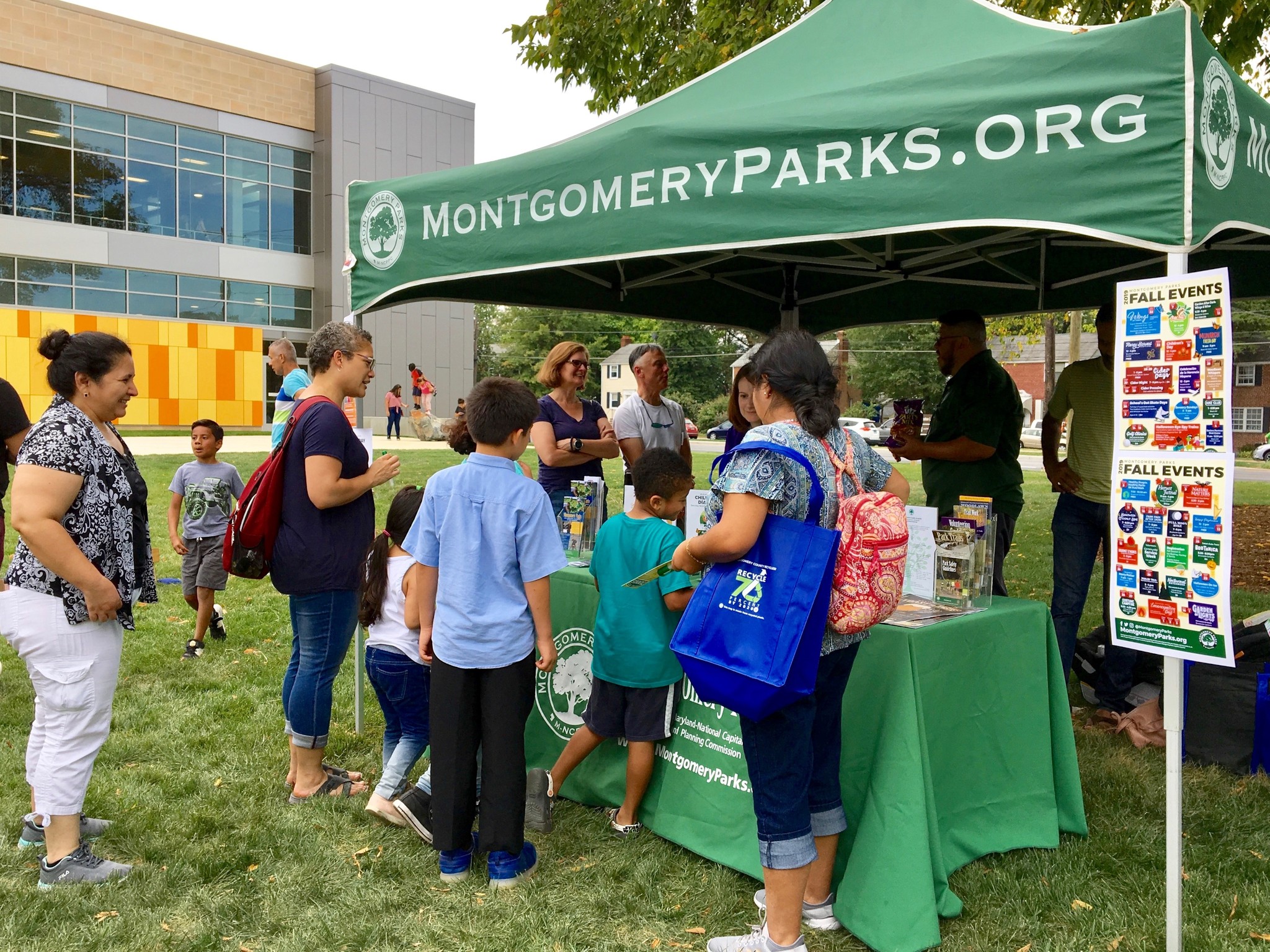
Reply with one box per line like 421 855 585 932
17 810 114 850
209 604 227 642
753 889 841 932
439 831 480 882
487 841 538 890
181 639 205 660
36 841 134 890
707 922 809 952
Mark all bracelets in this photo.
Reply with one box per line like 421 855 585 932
687 536 709 565
569 436 574 451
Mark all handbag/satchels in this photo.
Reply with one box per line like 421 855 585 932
1073 625 1162 711
1111 697 1165 749
667 440 840 713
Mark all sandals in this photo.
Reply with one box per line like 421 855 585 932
524 768 557 833
607 807 640 839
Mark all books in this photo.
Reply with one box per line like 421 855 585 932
929 494 994 610
560 475 603 559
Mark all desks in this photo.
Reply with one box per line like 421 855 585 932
523 549 1089 952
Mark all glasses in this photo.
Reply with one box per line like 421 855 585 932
341 349 376 372
565 359 591 370
641 399 674 428
935 335 971 345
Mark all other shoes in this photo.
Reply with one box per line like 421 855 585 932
387 434 390 439
413 403 421 412
365 793 408 830
392 787 433 844
397 435 400 440
425 411 431 416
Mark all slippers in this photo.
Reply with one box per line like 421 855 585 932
284 763 368 806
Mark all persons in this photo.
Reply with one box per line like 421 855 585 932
397 375 569 889
525 341 620 531
267 320 400 806
0 328 159 892
669 329 910 952
385 363 467 441
611 343 693 537
352 484 481 844
880 300 1026 598
0 378 33 571
1038 300 1119 686
166 418 247 662
268 337 313 456
520 448 695 839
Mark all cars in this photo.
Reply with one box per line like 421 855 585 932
1252 444 1270 462
1019 428 1066 451
706 420 733 441
684 418 699 439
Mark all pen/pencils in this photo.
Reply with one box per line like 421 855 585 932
382 450 394 488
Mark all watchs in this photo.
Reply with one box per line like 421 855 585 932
573 439 584 452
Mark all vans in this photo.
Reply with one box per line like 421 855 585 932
1030 419 1067 437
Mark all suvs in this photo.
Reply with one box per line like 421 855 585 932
838 417 881 447
877 418 894 446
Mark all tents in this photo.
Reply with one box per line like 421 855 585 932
342 0 1270 952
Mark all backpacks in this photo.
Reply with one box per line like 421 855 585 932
425 381 437 397
784 418 907 637
221 396 352 580
